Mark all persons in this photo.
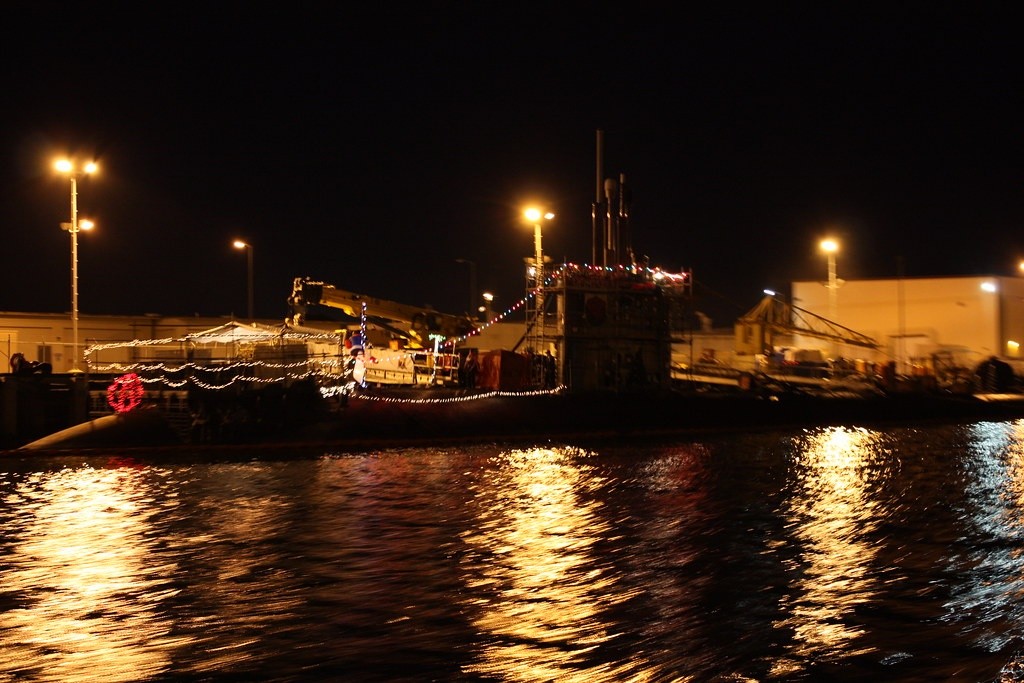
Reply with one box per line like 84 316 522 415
465 357 480 387
544 352 556 390
350 331 367 363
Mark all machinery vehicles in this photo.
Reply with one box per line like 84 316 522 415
284 276 482 384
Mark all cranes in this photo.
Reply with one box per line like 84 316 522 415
732 289 884 374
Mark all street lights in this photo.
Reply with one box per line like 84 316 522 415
524 208 555 355
233 240 254 325
54 157 99 374
